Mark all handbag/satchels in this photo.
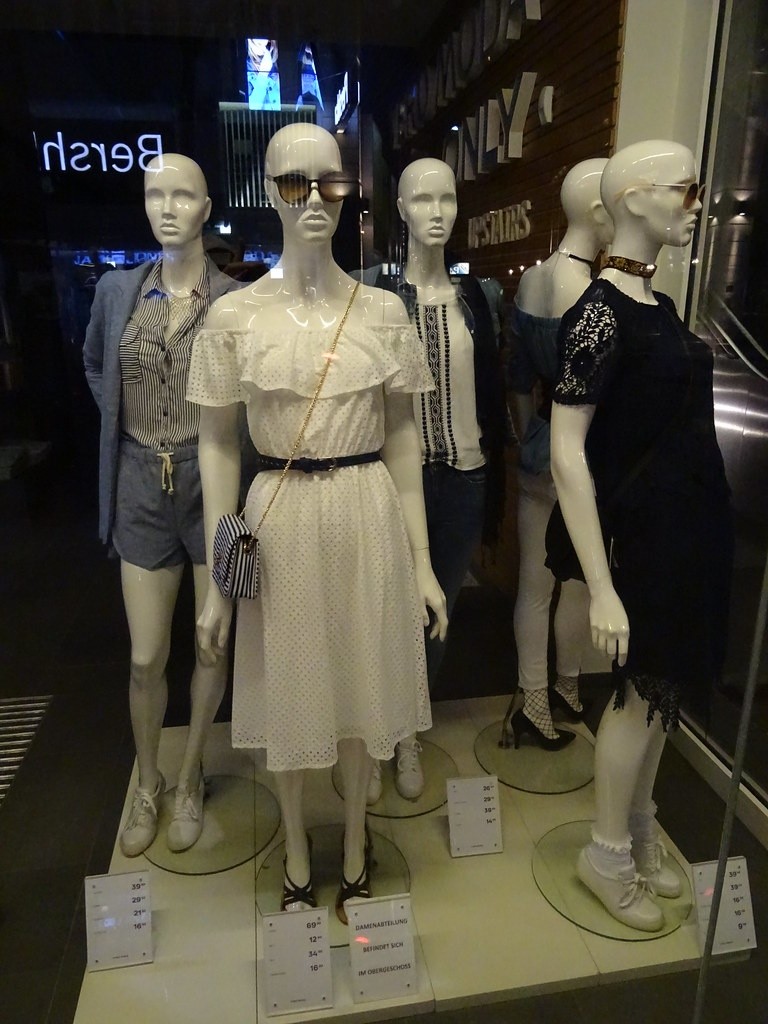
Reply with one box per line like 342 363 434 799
545 469 616 584
211 514 260 600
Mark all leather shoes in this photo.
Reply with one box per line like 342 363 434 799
576 847 665 931
631 833 680 898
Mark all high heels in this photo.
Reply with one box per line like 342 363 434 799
281 832 316 912
336 824 371 925
548 687 594 720
511 709 576 751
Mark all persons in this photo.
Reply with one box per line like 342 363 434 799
182 121 453 923
546 140 731 936
79 153 250 859
363 150 616 798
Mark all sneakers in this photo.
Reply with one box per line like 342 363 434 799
119 770 166 858
168 771 204 852
367 758 383 805
394 741 424 799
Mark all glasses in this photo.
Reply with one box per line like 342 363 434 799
266 172 355 205
615 182 706 209
